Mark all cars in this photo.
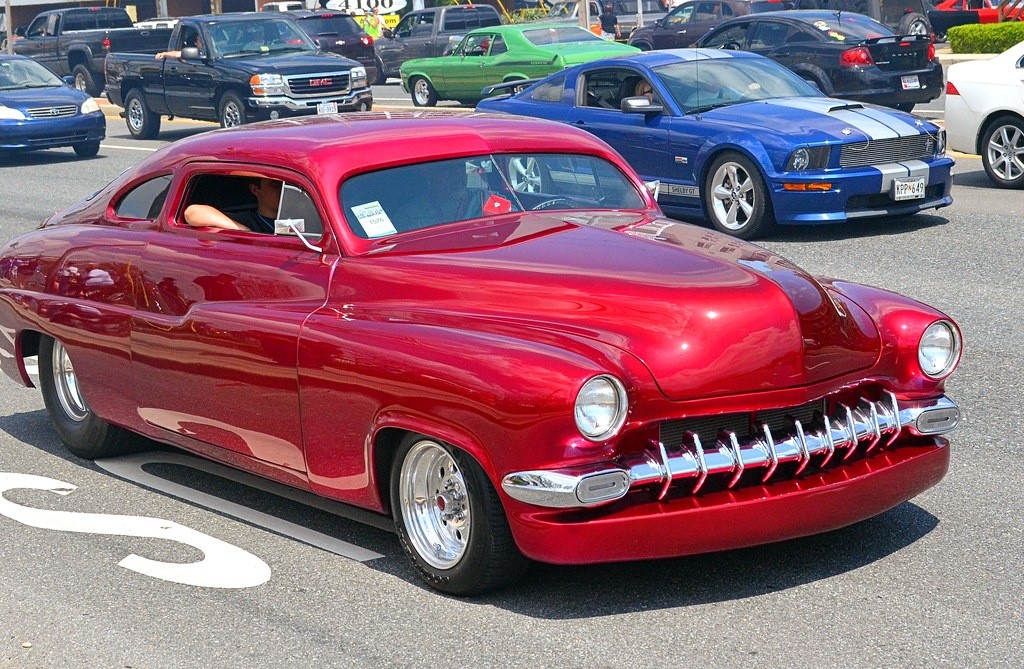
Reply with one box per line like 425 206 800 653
275 9 377 88
1 108 964 601
475 48 956 238
400 24 642 108
688 9 947 114
934 0 1024 23
548 0 670 44
0 56 106 157
945 40 1024 189
628 0 785 51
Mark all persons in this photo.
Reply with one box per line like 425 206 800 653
597 4 621 42
634 80 662 105
392 160 518 233
183 176 282 235
242 25 276 50
155 34 204 61
686 70 761 103
341 7 386 39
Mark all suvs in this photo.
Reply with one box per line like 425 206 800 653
749 1 932 40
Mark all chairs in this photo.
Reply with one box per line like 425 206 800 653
760 26 775 46
618 76 641 99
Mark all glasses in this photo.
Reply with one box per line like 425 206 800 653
643 88 652 95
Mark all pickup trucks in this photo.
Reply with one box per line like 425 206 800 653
368 4 560 86
0 8 176 98
103 13 373 141
